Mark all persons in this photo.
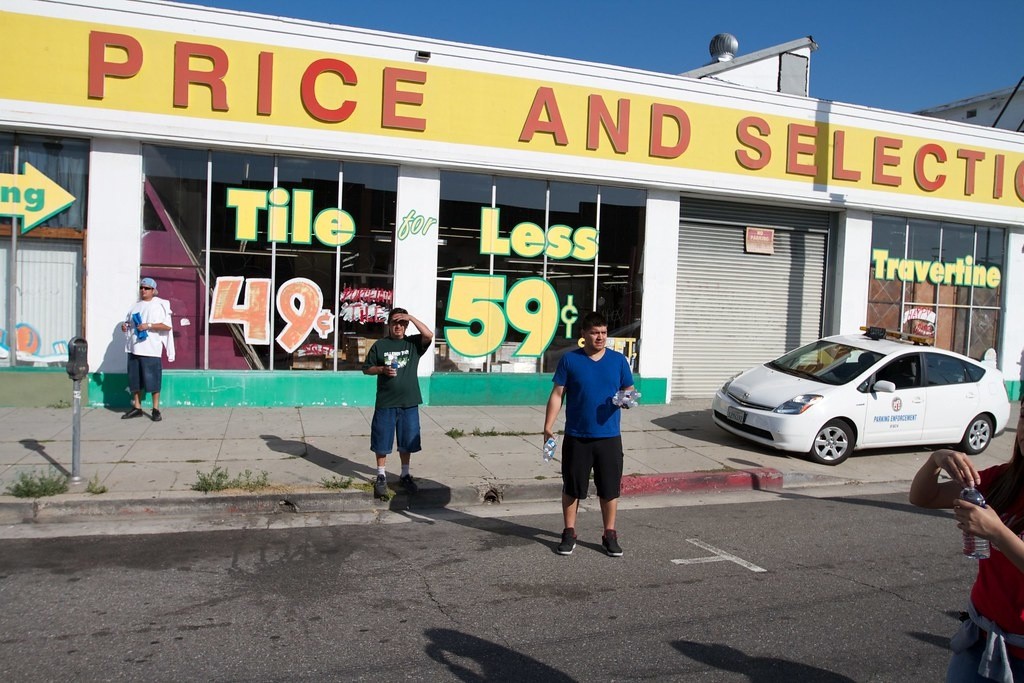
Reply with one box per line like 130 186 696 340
544 311 636 557
362 308 433 499
909 396 1024 683
121 277 176 422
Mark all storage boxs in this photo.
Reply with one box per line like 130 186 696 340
342 331 380 369
289 351 323 370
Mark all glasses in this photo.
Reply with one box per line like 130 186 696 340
140 286 154 290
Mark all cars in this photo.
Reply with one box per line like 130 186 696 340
711 332 1011 466
544 315 641 374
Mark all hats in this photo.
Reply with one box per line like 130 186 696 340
141 277 158 296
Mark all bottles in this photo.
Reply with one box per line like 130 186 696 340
616 390 642 400
391 356 398 371
543 446 557 461
124 323 132 343
543 433 560 452
613 395 638 407
959 482 989 560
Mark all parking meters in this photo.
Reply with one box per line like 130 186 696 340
66 338 90 483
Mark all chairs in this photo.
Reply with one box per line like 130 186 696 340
891 360 918 386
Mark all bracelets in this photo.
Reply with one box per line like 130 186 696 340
148 322 153 331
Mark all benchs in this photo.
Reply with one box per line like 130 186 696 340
925 357 965 384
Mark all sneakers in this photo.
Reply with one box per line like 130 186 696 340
398 473 418 493
122 408 142 419
372 474 390 499
556 527 577 554
601 529 623 556
151 408 162 421
